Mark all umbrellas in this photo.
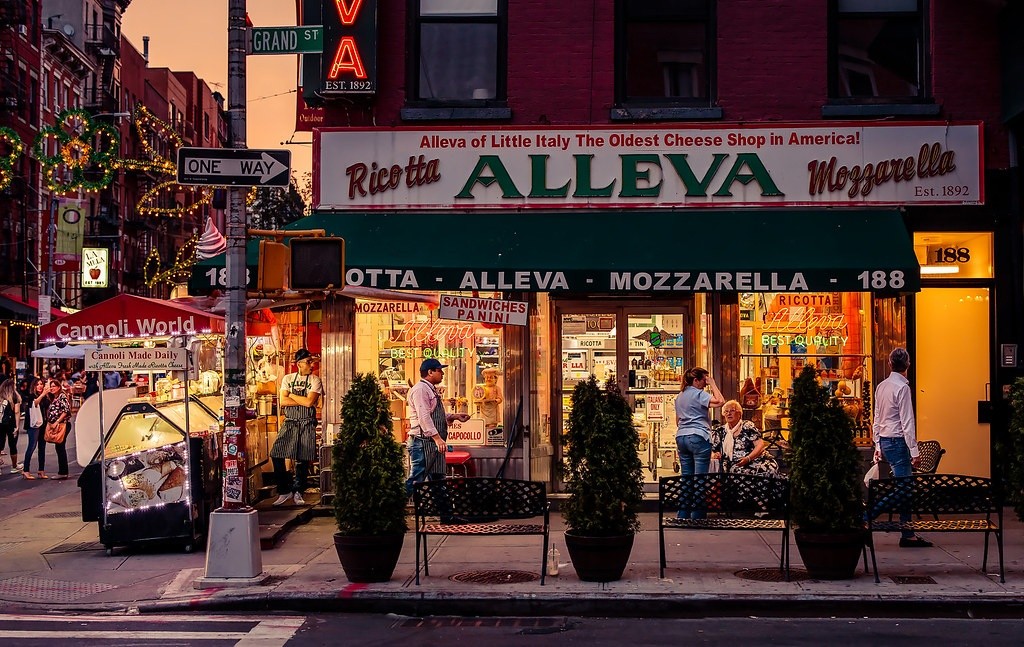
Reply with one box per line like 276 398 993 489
30 343 111 359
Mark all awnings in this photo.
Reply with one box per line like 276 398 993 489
188 210 921 299
0 295 72 320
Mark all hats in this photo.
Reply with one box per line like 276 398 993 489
419 359 448 371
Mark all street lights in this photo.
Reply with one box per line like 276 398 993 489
47 113 132 296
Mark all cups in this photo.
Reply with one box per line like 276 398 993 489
135 385 149 398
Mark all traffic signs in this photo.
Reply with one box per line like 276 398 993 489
176 146 292 188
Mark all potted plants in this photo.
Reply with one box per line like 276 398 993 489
563 376 646 582
330 372 410 582
785 364 868 581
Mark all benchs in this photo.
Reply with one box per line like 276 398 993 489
412 480 551 586
862 473 1005 584
658 475 793 575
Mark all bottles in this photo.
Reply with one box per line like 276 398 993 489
180 382 185 400
631 357 682 389
258 395 267 416
172 385 180 399
266 395 272 416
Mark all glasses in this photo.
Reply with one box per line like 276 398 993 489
299 360 315 364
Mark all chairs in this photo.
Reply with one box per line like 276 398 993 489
886 440 946 522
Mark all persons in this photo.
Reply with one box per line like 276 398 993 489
0 375 24 476
53 368 126 400
404 358 471 525
675 367 726 519
269 348 323 507
46 380 72 480
0 351 11 376
21 377 52 480
711 399 789 520
862 346 934 548
471 366 503 427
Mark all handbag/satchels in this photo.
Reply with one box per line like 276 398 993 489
863 462 879 489
29 401 43 429
43 422 66 444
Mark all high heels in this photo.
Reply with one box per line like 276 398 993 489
38 472 48 478
21 473 35 480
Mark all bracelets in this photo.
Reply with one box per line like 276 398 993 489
745 455 751 463
56 418 60 423
41 394 45 398
286 392 291 397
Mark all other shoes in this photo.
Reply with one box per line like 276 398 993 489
272 492 293 507
439 515 469 525
899 534 934 547
50 473 68 480
754 508 769 518
10 463 24 474
293 491 305 506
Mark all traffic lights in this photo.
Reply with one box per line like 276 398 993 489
289 237 345 292
